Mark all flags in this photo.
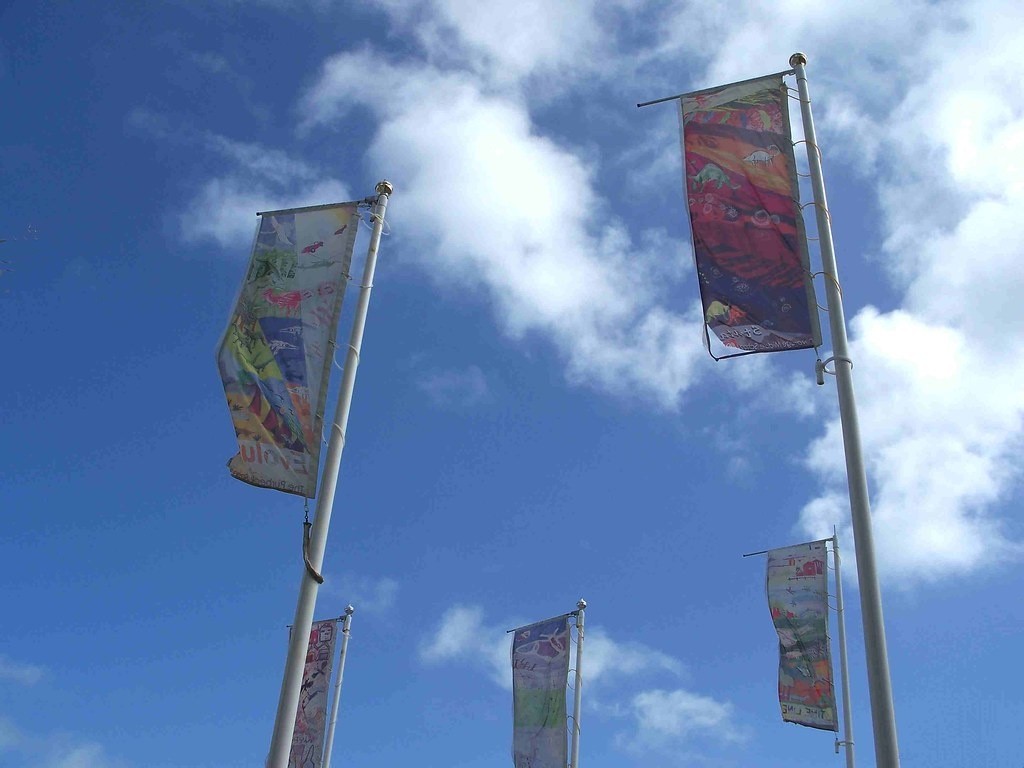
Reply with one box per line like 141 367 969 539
768 539 839 732
286 619 336 768
680 75 820 360
511 615 571 768
217 202 358 499
